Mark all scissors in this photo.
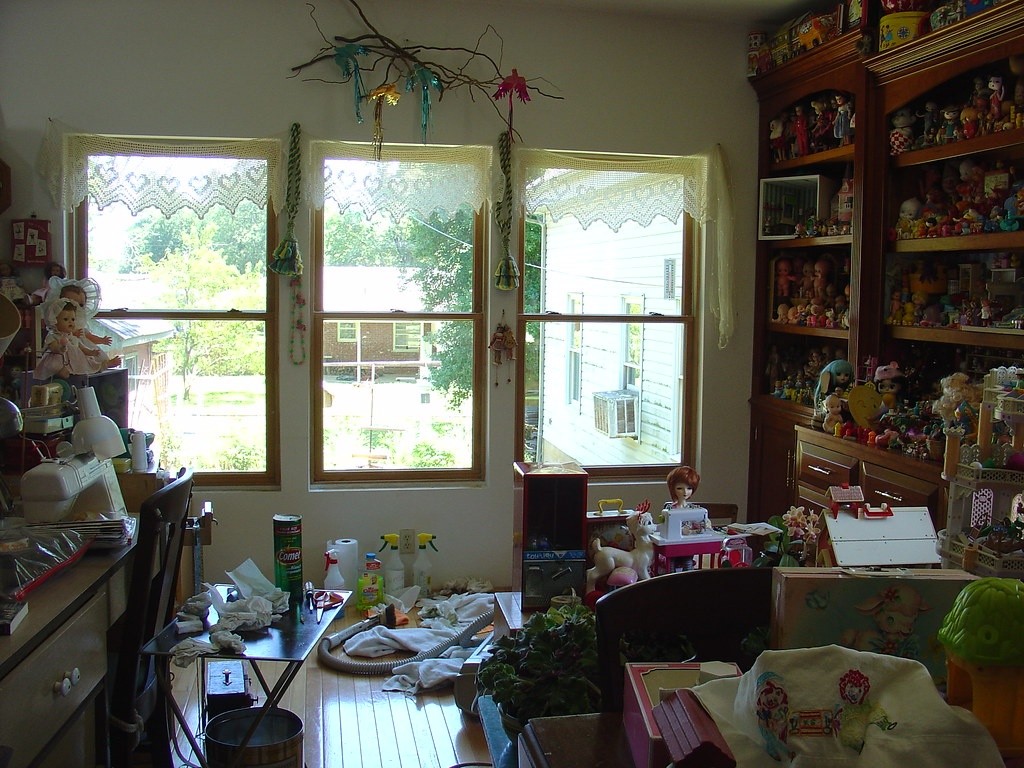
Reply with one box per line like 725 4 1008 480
313 591 344 624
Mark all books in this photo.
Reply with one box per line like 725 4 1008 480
0 599 30 635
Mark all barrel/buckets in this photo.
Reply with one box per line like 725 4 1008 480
203 707 304 768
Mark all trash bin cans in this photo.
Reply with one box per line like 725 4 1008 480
204 706 306 768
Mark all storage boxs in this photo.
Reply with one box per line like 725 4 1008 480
770 563 983 704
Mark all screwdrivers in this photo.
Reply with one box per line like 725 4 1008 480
305 580 315 611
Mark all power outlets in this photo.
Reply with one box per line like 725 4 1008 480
399 529 415 554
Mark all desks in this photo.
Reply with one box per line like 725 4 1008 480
517 712 636 768
140 584 354 768
493 592 534 639
476 695 512 768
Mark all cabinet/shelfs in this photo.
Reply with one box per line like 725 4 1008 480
115 458 219 619
752 28 870 419
792 424 951 532
0 514 140 768
747 396 811 525
863 0 1023 365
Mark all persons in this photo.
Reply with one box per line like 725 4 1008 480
658 466 711 575
24 262 66 306
761 54 1024 436
0 261 30 303
32 297 108 377
43 275 122 373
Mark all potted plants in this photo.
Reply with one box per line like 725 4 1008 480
474 603 602 750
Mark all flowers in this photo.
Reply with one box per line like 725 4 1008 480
769 505 822 566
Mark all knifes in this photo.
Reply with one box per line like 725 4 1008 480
316 600 324 623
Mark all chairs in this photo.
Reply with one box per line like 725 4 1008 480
663 503 739 528
96 466 198 768
594 566 774 714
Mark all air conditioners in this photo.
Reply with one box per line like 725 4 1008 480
592 390 638 437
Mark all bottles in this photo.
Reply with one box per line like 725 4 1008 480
723 536 752 568
356 553 384 612
